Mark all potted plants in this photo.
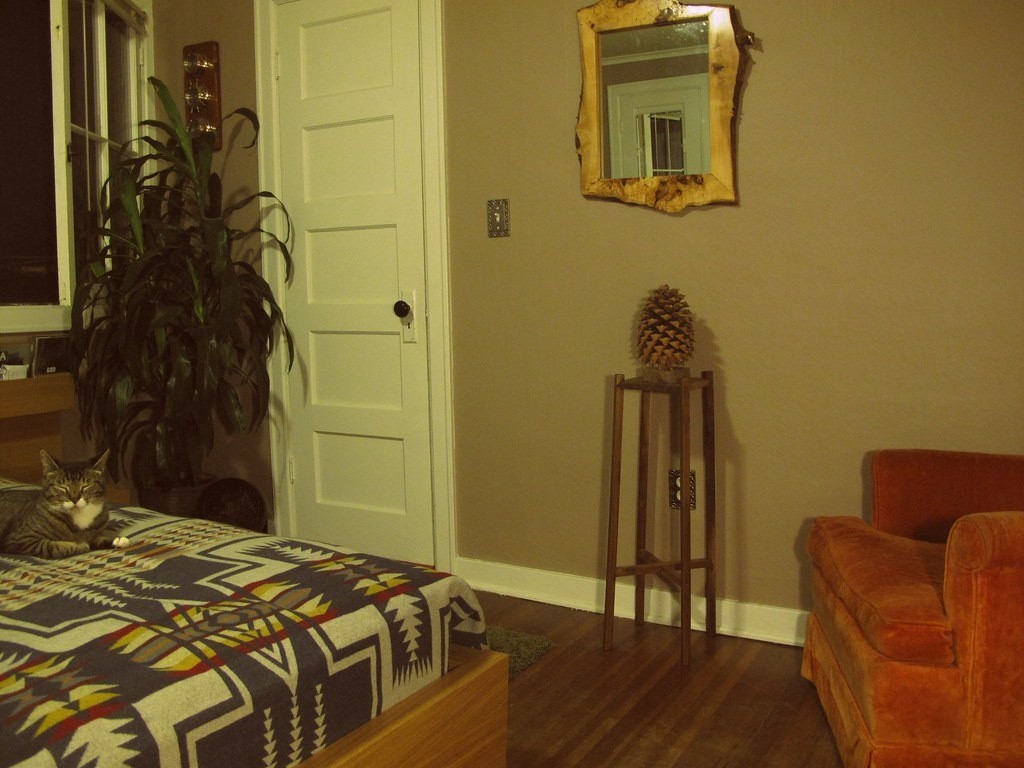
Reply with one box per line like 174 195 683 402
68 76 292 533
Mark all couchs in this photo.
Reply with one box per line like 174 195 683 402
799 447 1024 768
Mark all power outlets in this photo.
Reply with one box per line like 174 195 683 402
668 469 697 510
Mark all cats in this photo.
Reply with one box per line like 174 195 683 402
0 448 130 557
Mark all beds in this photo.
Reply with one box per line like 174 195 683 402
0 477 511 768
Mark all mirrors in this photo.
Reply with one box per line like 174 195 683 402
573 0 756 214
